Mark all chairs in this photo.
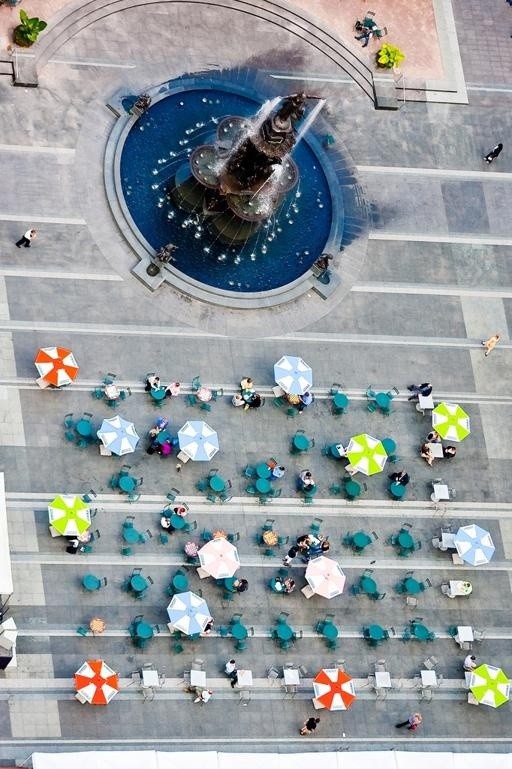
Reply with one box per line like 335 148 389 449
353 9 389 42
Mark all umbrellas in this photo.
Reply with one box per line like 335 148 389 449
429 400 472 441
75 659 119 706
47 494 91 538
313 668 356 711
33 346 78 387
468 664 511 709
271 355 313 396
454 523 496 567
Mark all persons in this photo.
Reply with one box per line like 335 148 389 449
483 143 504 165
16 228 36 249
66 542 78 555
408 382 432 401
174 507 186 517
202 616 214 633
395 712 422 731
298 716 322 735
146 416 173 458
278 91 306 123
388 468 409 487
285 535 330 593
191 687 212 705
288 390 312 416
481 333 501 356
438 537 449 551
223 659 238 688
144 376 180 399
461 654 476 672
161 516 175 535
354 24 374 48
237 579 248 593
176 463 181 472
233 377 261 407
270 466 314 493
421 431 456 467
